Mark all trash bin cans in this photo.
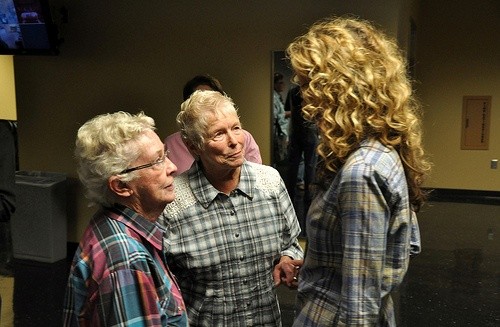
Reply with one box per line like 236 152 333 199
10 170 77 263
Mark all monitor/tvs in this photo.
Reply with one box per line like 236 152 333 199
0 0 67 57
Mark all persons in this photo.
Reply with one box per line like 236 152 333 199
295 150 307 190
282 12 434 326
153 89 304 327
273 72 292 170
284 86 314 216
61 111 190 327
162 73 263 178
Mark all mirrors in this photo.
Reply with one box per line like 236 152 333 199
270 49 327 240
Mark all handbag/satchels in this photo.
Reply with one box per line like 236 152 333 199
0 274 14 327
272 118 287 162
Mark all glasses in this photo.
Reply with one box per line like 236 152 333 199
117 147 169 177
275 81 284 85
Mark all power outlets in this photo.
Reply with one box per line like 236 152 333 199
491 160 498 169
